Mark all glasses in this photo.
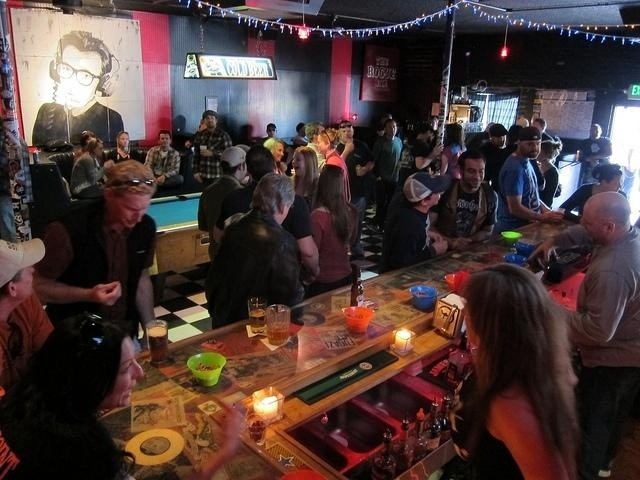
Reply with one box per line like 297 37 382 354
124 178 155 186
56 64 100 86
79 311 105 349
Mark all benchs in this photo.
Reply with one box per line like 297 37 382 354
24 140 185 249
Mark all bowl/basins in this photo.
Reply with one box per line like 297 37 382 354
445 271 468 290
341 306 377 334
502 225 536 266
186 351 228 388
410 285 437 310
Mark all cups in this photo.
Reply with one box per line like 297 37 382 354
200 145 207 152
145 319 169 366
247 297 292 346
246 414 269 446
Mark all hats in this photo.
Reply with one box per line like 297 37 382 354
220 146 246 168
490 124 507 136
518 126 541 140
0 238 46 288
403 172 452 202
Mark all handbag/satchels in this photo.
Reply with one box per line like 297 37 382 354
43 139 73 152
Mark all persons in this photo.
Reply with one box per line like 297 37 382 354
548 192 639 480
30 30 123 139
1 238 59 395
1 111 640 347
12 316 245 478
443 262 579 480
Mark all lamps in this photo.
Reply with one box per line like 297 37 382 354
297 0 310 41
182 0 278 81
501 8 512 62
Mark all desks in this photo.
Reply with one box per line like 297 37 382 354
144 191 211 277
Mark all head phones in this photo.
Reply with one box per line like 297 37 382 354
49 45 120 97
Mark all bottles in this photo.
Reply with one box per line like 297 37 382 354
543 249 568 289
371 395 453 480
350 266 366 306
